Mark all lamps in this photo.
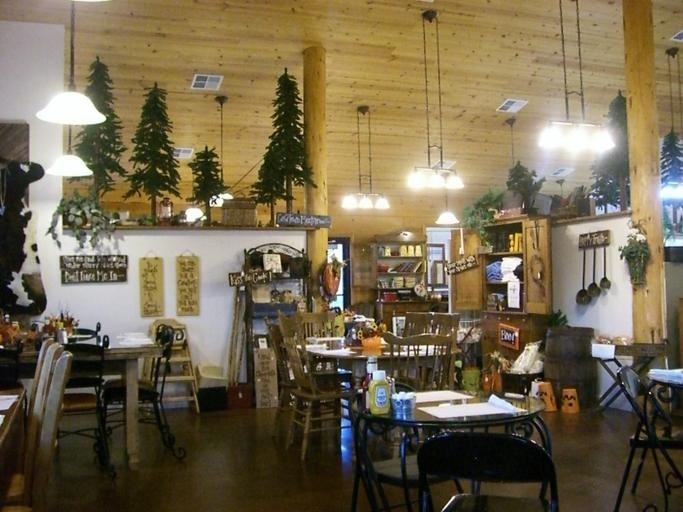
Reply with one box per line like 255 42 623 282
401 231 412 241
35 0 107 178
436 195 460 225
502 118 529 212
658 36 683 204
210 93 234 208
538 1 614 153
185 162 208 222
342 103 389 210
408 12 464 189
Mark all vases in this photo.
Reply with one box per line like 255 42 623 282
363 338 380 356
57 329 68 345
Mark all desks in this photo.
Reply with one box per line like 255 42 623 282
592 343 672 426
1 333 164 472
348 392 551 512
0 386 27 511
292 337 466 455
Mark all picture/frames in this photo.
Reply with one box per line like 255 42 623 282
393 316 405 336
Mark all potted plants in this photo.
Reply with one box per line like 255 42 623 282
506 160 546 215
459 206 494 254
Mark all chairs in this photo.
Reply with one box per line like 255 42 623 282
348 381 464 512
423 313 462 391
32 323 117 480
277 309 372 461
593 356 683 512
93 324 187 474
28 336 54 432
384 328 456 398
390 308 432 384
16 344 65 473
416 432 558 511
264 308 346 440
4 352 72 512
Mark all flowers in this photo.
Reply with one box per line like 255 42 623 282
44 313 77 333
353 319 386 338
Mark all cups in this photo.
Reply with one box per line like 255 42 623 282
403 395 416 416
391 395 402 415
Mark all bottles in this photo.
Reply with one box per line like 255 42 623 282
362 356 377 412
160 198 173 227
369 371 390 415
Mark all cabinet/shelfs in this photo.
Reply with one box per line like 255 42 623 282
370 227 435 333
448 207 552 395
244 242 312 403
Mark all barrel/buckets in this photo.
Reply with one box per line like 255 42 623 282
543 326 598 410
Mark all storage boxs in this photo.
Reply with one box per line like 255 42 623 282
198 387 226 411
228 384 249 407
384 291 397 300
251 278 304 303
398 290 410 300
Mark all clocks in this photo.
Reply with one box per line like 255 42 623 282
262 253 282 273
